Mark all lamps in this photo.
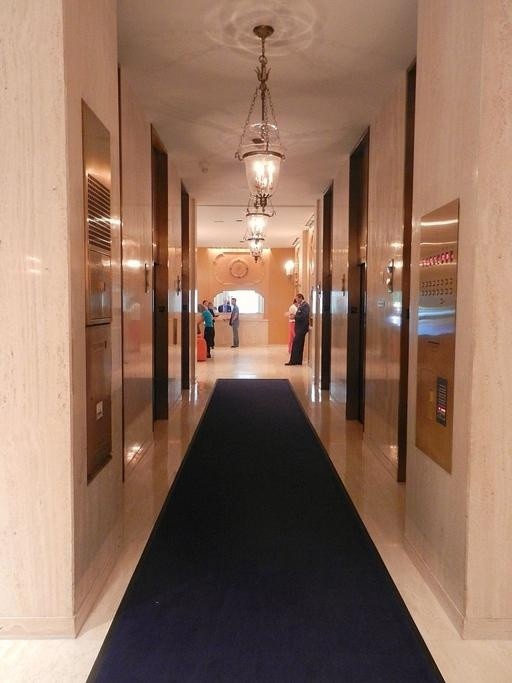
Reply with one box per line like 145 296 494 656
236 25 285 263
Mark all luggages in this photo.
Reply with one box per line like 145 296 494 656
197 333 207 361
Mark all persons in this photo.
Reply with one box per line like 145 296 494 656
288 297 298 353
284 293 310 366
196 296 240 358
